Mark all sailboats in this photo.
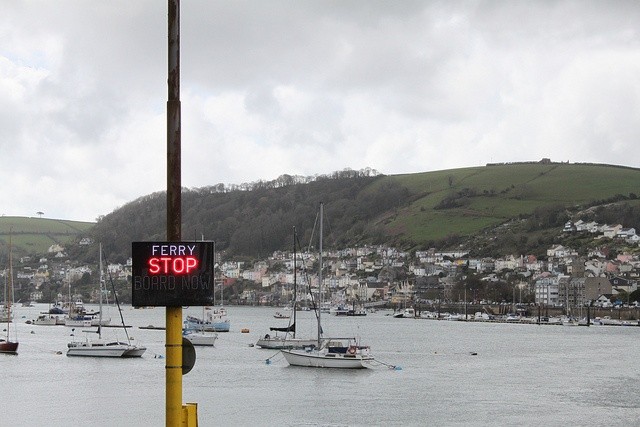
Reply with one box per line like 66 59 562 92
0 265 13 322
67 241 146 356
182 233 218 345
256 224 323 349
184 278 230 331
280 200 374 368
0 227 18 354
34 276 102 325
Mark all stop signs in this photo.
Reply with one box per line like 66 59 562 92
131 240 215 306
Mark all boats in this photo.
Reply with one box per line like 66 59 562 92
274 311 290 318
